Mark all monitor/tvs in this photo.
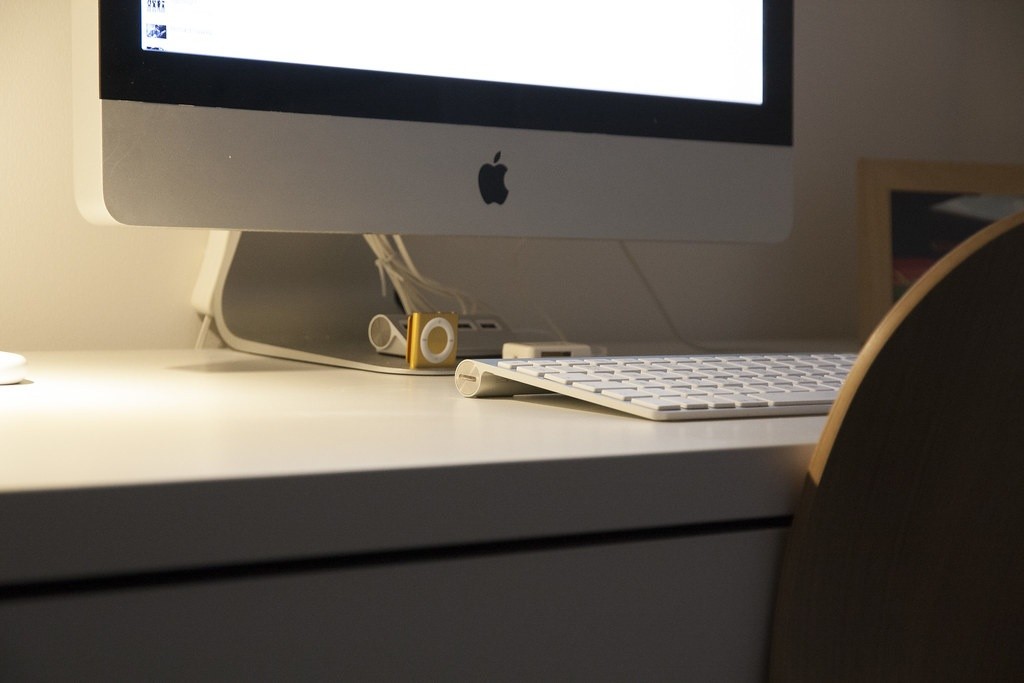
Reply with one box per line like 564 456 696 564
76 0 795 375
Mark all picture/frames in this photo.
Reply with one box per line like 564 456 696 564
854 157 1024 354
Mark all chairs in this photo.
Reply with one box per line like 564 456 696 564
760 211 1024 683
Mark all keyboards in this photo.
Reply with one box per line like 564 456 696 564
454 353 859 421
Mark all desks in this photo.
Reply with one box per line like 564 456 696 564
0 341 856 683
